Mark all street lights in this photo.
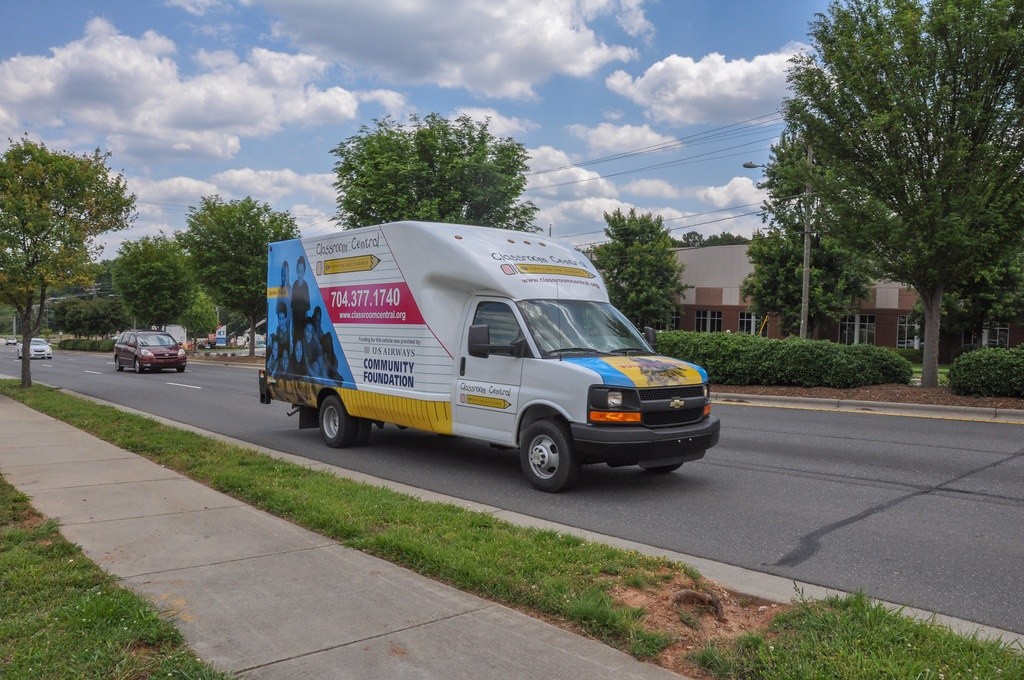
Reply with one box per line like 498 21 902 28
741 146 813 339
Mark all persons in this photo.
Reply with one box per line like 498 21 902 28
267 256 344 387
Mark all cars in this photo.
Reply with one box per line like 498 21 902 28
5 336 19 346
17 337 53 360
114 328 188 374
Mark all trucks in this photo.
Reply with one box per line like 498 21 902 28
258 220 721 494
